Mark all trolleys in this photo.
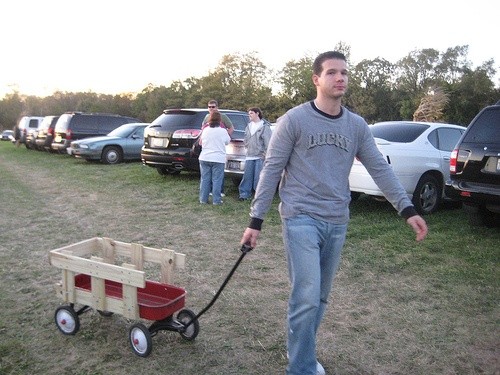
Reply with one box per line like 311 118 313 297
47 233 255 358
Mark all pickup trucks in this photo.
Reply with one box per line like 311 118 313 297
224 121 278 188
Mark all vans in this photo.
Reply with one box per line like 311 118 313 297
33 116 60 151
50 112 145 156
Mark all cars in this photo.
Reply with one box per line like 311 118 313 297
348 119 468 216
65 121 152 165
0 129 14 141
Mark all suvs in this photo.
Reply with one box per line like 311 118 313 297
12 114 45 150
443 103 500 229
140 107 253 179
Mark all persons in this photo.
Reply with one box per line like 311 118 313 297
201 100 234 197
198 110 231 205
238 108 272 202
240 51 428 375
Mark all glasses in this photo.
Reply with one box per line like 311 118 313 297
208 106 216 108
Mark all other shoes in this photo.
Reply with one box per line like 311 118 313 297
209 192 212 196
220 192 226 197
287 350 325 375
